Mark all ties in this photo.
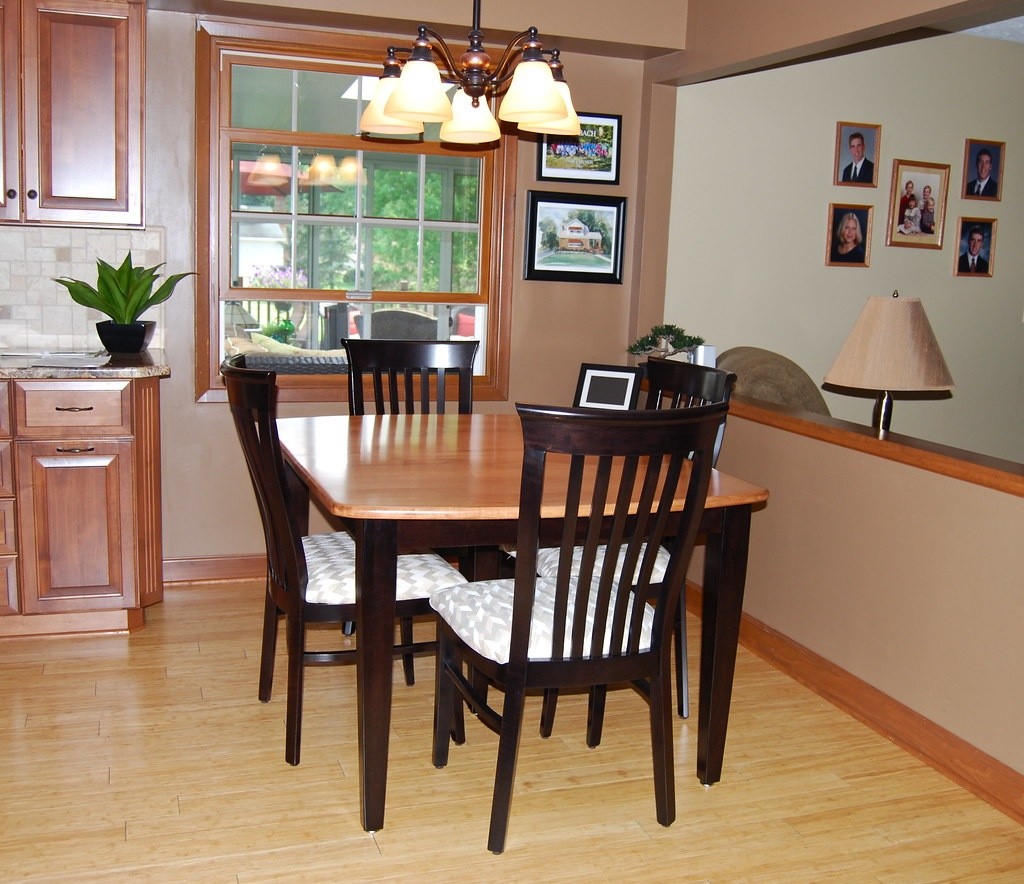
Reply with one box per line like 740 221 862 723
975 183 981 195
971 257 978 273
851 165 859 182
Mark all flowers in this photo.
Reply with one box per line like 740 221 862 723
249 264 308 289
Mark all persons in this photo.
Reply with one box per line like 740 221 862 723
831 212 865 263
966 148 997 198
958 225 989 273
897 180 935 234
842 132 874 184
550 143 610 158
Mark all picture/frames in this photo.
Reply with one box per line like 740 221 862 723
954 216 998 278
885 158 951 250
536 111 622 186
960 138 1007 202
522 189 629 285
825 203 874 268
833 121 882 188
572 362 644 410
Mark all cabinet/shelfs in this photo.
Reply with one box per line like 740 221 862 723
0 377 165 642
0 0 148 232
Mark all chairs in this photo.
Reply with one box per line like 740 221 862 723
502 356 737 736
429 401 730 856
221 352 468 768
716 345 831 419
342 338 480 687
353 308 453 341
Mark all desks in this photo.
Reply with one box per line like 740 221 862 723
254 413 769 833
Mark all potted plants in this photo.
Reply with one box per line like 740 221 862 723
49 251 201 352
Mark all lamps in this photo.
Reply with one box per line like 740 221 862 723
247 154 368 187
224 300 259 338
358 0 581 143
822 289 956 432
